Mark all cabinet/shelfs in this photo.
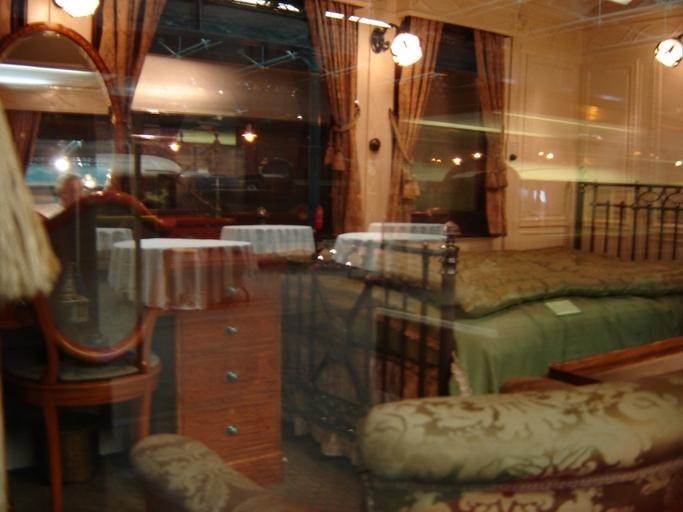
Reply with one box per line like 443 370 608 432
169 254 284 485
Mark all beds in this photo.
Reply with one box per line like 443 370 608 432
281 177 682 465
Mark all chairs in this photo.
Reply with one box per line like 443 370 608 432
132 378 683 512
0 192 176 510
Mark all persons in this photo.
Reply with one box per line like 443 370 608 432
32 172 90 221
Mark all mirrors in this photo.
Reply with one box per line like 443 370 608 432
0 22 127 221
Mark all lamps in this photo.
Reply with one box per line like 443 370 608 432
371 22 422 68
55 0 100 19
653 33 683 69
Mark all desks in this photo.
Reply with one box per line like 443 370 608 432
94 228 133 252
553 339 683 385
110 237 258 311
220 225 315 269
334 220 447 284
